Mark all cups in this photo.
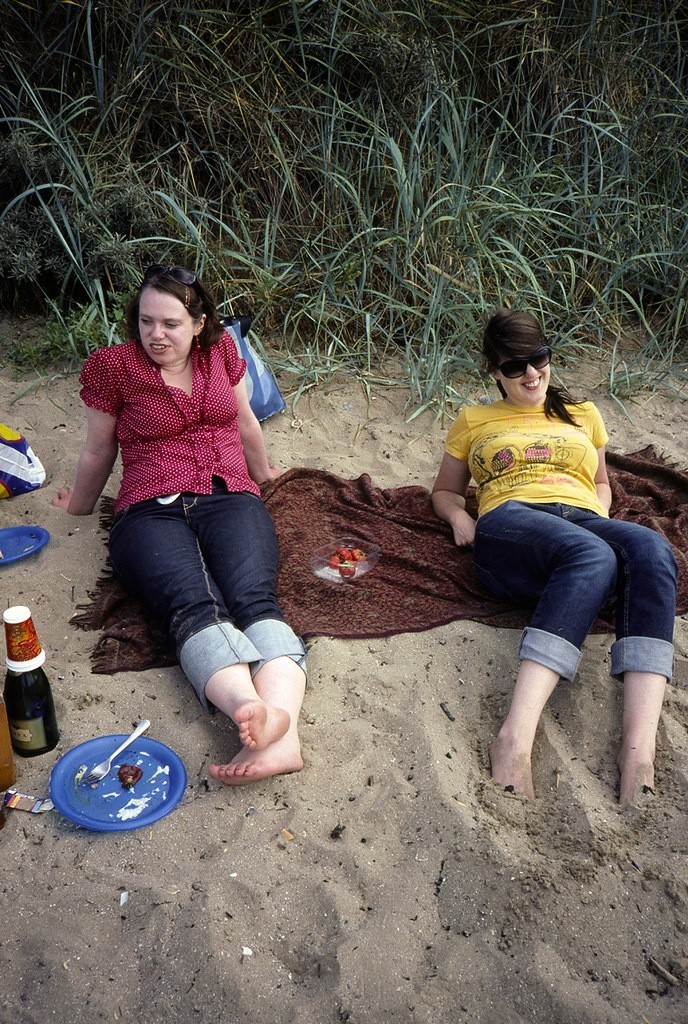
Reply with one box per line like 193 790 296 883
2 606 46 672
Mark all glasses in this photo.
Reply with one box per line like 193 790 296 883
496 346 553 379
144 264 205 298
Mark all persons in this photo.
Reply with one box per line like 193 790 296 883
430 308 679 810
51 264 306 786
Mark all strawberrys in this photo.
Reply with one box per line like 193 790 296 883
327 548 367 579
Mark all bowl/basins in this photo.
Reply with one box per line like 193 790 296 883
310 537 381 584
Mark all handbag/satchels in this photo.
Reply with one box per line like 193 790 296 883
0 423 46 499
216 316 287 423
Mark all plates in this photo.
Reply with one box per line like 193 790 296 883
0 525 50 564
48 734 187 831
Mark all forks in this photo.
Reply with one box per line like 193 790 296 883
84 719 151 784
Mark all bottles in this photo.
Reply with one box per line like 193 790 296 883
0 688 17 793
3 667 60 758
